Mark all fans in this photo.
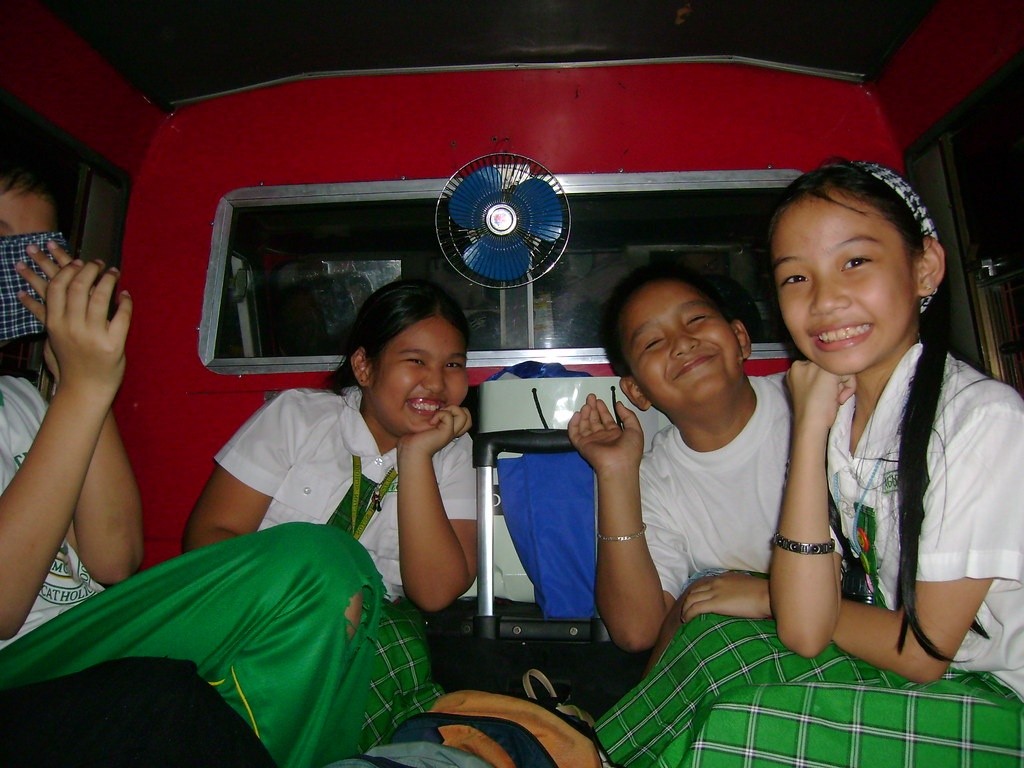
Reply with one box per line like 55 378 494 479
435 152 572 289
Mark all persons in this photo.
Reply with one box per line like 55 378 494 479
0 146 387 768
567 259 793 653
179 277 478 753
592 156 1024 768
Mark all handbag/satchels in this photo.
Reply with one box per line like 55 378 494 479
323 670 610 768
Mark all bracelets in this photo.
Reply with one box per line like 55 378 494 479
772 532 836 555
597 522 646 541
